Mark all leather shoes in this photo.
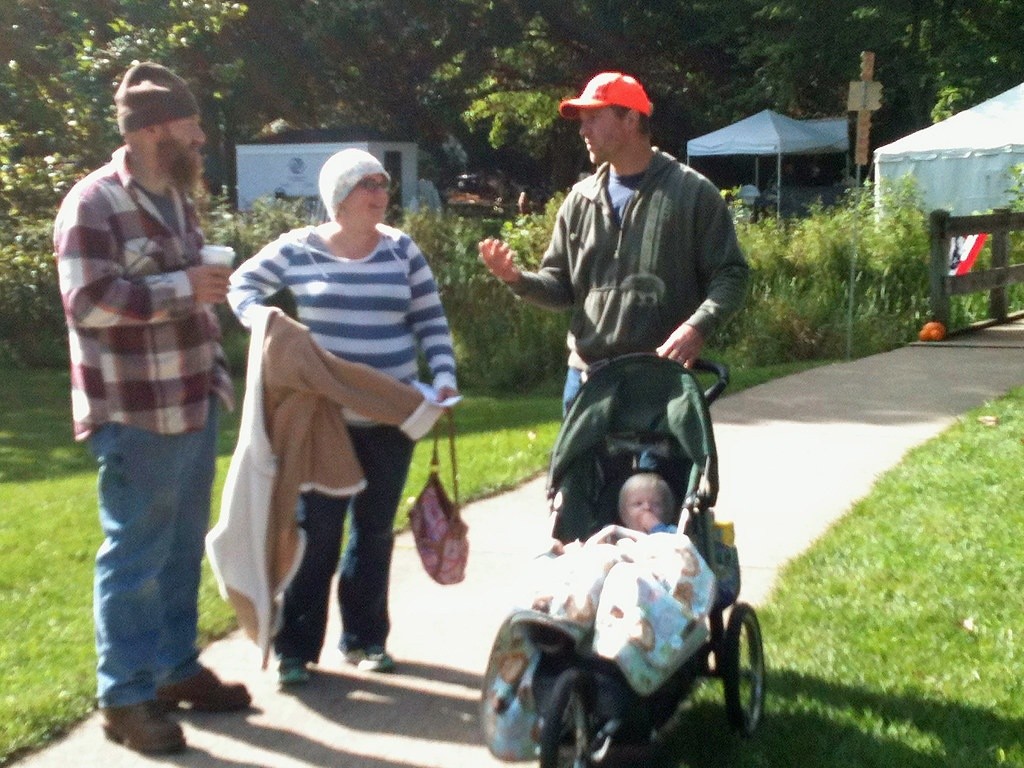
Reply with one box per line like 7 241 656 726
95 695 188 756
153 665 251 711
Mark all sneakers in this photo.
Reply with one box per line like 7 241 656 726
273 655 310 684
335 634 393 670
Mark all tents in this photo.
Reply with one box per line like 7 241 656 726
873 81 1024 237
683 109 850 222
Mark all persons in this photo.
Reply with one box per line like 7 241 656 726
225 147 461 685
531 471 682 660
477 72 751 472
52 63 252 755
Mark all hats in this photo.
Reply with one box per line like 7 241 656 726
557 69 655 119
316 147 391 222
112 61 199 133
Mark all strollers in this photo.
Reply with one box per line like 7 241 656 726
524 350 769 768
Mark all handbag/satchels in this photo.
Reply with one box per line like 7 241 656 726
407 403 467 583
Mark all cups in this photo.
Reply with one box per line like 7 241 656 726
199 243 237 267
